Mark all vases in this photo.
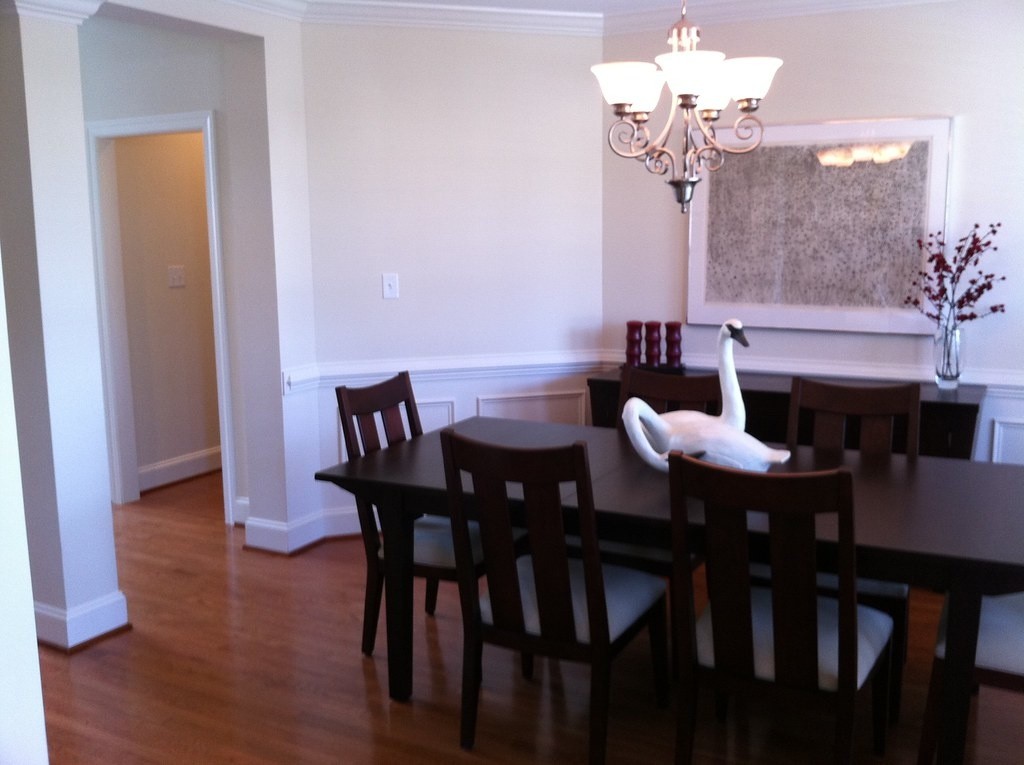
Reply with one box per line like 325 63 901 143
934 327 962 390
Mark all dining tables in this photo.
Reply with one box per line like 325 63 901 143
314 414 1024 765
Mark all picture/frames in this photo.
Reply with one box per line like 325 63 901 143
686 118 952 335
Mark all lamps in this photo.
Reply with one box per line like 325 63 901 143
591 0 784 215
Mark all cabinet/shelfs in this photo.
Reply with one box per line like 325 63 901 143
587 363 987 458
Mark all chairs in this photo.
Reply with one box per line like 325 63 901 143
918 590 1024 764
335 370 528 655
438 427 668 765
566 364 722 707
747 375 922 728
669 450 894 765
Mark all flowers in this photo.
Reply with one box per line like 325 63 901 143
904 221 1006 327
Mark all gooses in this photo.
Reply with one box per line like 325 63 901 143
622 317 792 477
